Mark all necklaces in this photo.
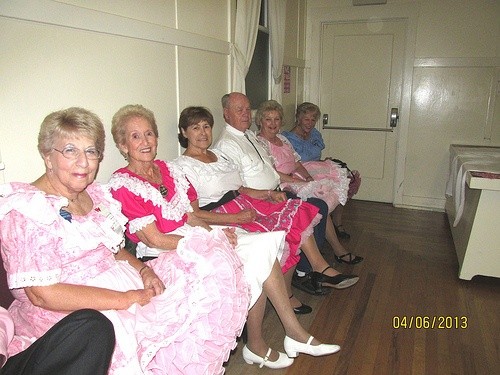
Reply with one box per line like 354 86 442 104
44 172 77 202
128 164 169 196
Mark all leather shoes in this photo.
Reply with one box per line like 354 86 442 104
283 335 341 357
242 345 294 369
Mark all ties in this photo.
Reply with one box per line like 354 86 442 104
244 133 265 164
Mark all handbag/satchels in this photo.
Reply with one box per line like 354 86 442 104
332 159 355 183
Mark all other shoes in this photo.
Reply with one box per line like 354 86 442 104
333 223 350 238
292 270 330 295
335 253 364 265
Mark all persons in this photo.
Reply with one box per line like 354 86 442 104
103 104 342 369
0 308 116 375
253 100 364 264
214 92 330 295
281 101 351 239
172 107 360 314
0 106 251 375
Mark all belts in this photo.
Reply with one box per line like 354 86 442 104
199 190 240 212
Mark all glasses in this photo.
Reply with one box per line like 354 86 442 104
53 146 101 159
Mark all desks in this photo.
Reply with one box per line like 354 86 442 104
445 144 500 280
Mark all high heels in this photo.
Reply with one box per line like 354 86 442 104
289 295 312 314
311 266 360 292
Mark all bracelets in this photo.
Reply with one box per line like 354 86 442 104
292 178 297 183
268 189 273 197
306 176 311 179
140 266 153 275
